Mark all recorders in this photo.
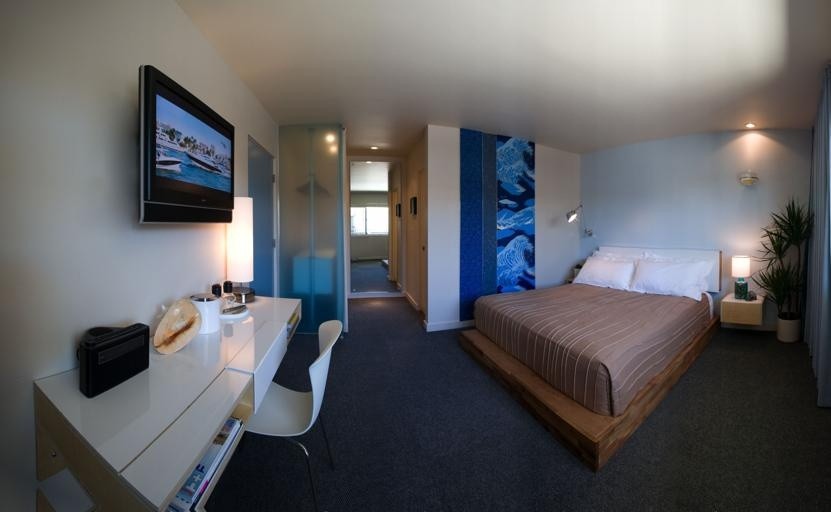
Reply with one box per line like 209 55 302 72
79 322 149 398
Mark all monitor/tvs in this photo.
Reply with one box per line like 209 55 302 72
139 65 234 224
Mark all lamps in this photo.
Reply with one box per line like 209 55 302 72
566 202 596 240
731 255 753 299
737 168 761 189
227 194 259 303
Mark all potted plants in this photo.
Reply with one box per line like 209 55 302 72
752 195 816 345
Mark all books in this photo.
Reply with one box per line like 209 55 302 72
167 416 243 512
287 311 300 339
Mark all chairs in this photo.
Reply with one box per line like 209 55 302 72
241 317 346 512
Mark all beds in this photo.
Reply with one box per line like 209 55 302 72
456 245 723 472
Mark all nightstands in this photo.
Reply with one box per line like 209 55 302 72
720 291 769 327
563 275 575 284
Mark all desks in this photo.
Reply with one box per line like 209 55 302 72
30 295 306 512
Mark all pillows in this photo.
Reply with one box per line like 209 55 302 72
570 248 716 305
